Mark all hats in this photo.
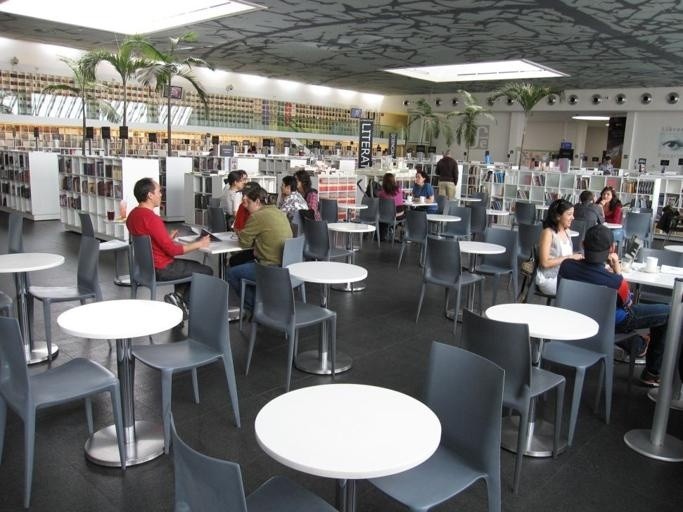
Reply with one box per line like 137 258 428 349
583 224 613 263
234 182 260 195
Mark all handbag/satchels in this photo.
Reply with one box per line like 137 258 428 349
520 246 535 277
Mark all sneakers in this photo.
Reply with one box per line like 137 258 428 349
164 293 187 320
635 340 649 357
638 367 661 387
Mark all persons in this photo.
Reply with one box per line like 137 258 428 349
126 178 212 321
434 148 459 199
661 138 681 150
411 171 439 214
556 225 671 387
227 187 293 322
598 157 615 176
219 168 323 237
376 173 404 241
595 187 621 241
533 199 586 297
572 191 605 240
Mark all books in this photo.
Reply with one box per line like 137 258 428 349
58 158 122 210
469 170 681 243
193 159 218 225
159 157 166 216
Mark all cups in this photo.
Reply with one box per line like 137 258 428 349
620 256 634 273
644 256 659 273
